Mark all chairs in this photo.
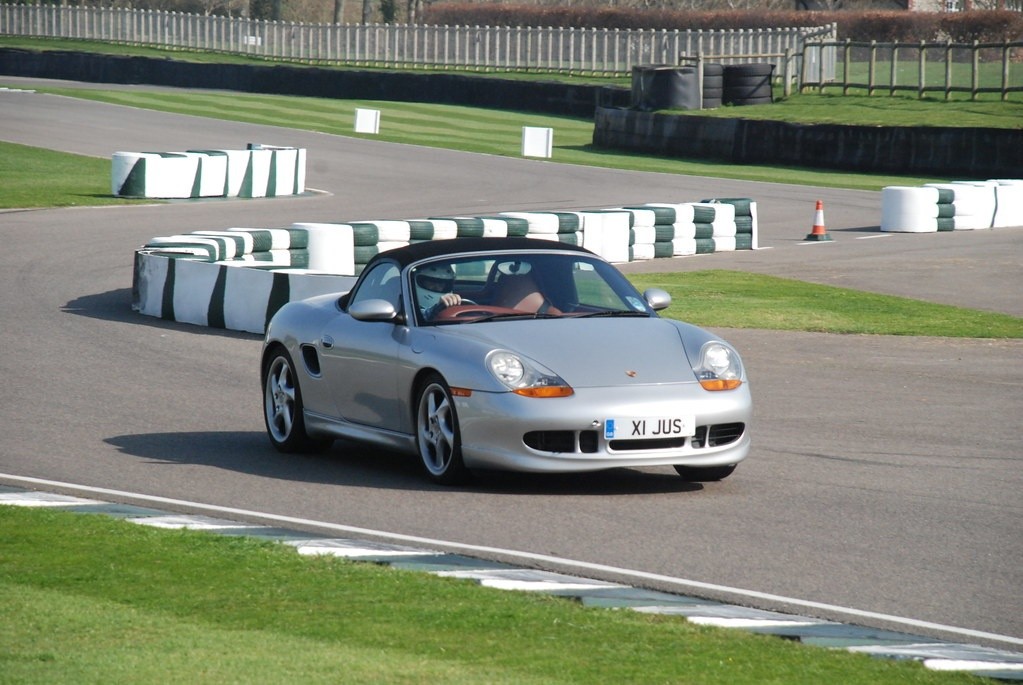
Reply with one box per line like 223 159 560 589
485 274 561 315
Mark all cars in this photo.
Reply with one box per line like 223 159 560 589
260 236 752 487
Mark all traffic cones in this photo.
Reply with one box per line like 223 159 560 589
802 199 833 241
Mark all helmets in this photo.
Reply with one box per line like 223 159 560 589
412 264 455 308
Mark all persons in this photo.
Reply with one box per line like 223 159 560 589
415 261 462 321
